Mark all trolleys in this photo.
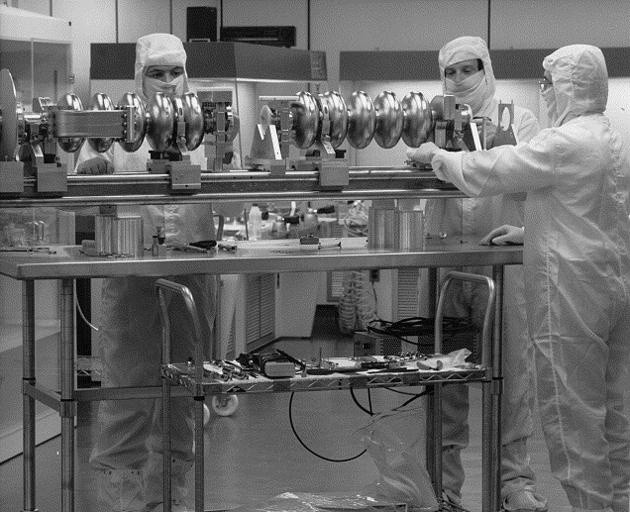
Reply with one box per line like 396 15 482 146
200 272 239 429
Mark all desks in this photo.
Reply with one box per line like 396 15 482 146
2 232 523 511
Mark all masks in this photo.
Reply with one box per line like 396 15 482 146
445 70 484 97
543 90 557 118
143 76 185 96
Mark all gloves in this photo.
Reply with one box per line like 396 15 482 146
414 141 438 164
481 224 524 246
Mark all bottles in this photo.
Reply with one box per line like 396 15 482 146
248 203 339 239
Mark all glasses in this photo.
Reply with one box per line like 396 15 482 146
539 81 553 91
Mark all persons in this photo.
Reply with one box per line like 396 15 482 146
72 33 222 512
395 37 549 510
404 44 629 512
